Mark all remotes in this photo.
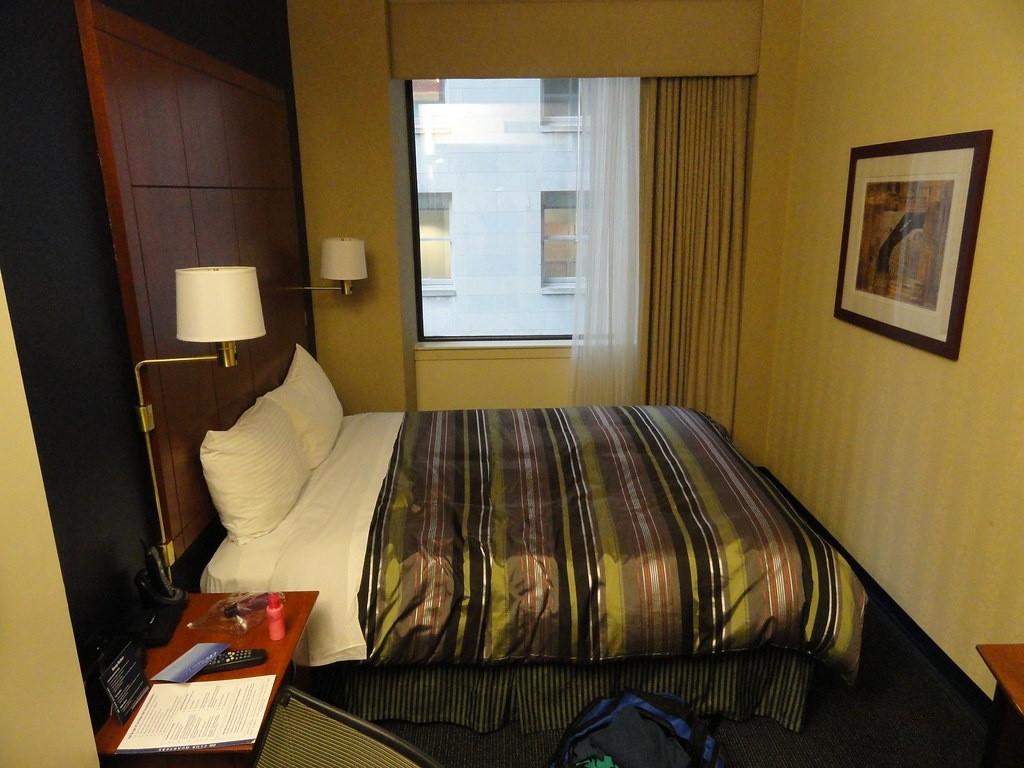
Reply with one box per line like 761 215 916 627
198 648 267 675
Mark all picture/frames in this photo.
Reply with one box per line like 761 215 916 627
833 129 994 361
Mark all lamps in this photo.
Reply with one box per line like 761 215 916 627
305 238 368 296
135 265 268 585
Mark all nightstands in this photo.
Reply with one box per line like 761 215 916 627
93 591 319 768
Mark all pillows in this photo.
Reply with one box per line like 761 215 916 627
199 395 312 543
264 343 343 470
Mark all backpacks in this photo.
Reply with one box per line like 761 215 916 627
542 691 732 768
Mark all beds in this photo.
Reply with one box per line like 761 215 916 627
200 405 871 738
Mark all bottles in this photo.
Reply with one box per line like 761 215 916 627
266 596 285 641
225 592 269 617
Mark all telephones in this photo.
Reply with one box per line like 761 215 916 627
135 537 189 607
